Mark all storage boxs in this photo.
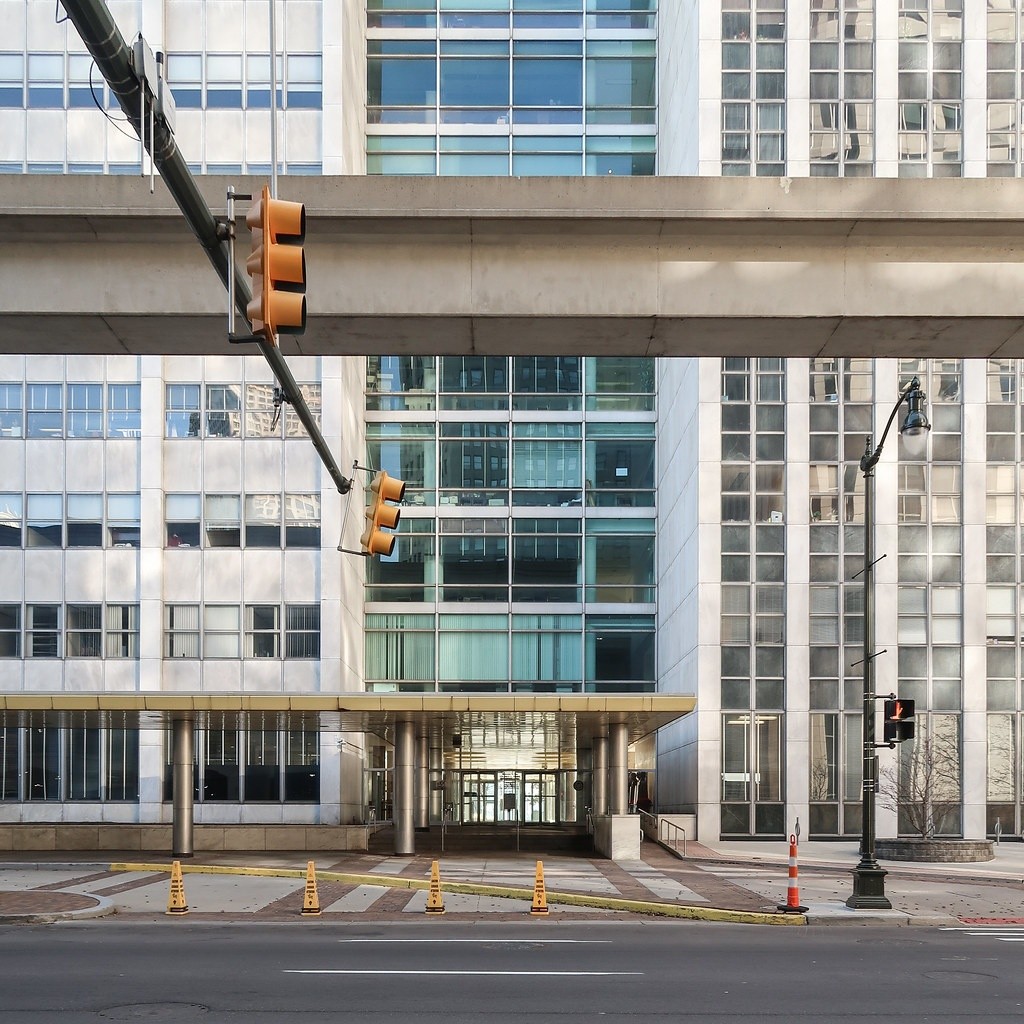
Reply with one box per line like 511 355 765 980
450 496 458 503
440 503 456 506
11 427 22 437
440 497 448 503
489 499 504 505
411 504 421 507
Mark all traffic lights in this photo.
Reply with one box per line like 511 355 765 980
360 470 405 557
884 699 916 743
246 185 307 348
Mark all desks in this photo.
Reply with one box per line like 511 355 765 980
2 428 30 436
87 430 112 437
496 597 506 600
547 596 560 602
396 597 411 601
519 597 535 602
116 429 141 437
470 597 483 601
40 428 62 432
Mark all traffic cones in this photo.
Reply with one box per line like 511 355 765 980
530 861 549 916
165 860 189 916
425 860 446 915
300 861 322 917
777 833 809 913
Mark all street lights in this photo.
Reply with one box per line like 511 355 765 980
845 374 932 909
